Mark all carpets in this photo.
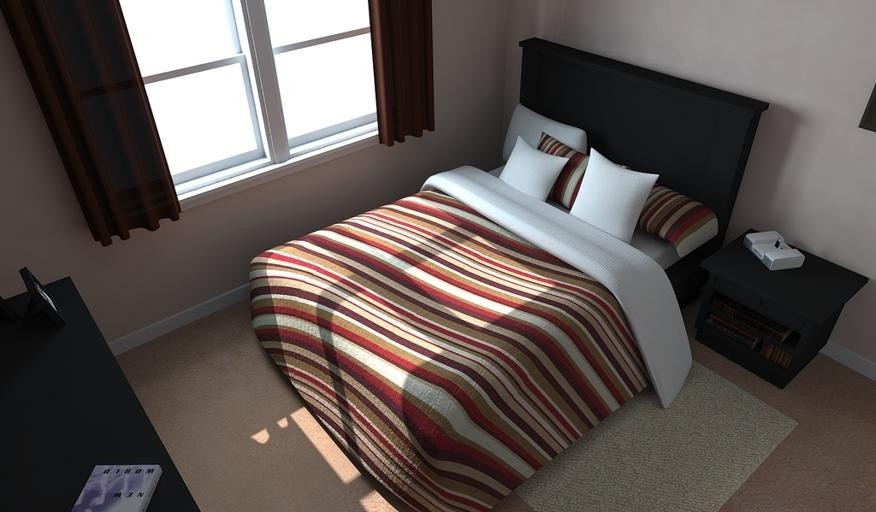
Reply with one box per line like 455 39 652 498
512 357 799 512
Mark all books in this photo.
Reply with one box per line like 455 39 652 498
70 464 163 512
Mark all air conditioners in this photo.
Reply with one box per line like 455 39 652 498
502 105 589 167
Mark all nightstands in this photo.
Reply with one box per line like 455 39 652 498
696 227 869 390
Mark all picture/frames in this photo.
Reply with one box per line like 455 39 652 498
18 266 68 329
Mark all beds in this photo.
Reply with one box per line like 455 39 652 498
249 35 768 512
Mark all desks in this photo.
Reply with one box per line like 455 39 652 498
0 275 200 512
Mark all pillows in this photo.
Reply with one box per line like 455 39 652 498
533 133 593 211
498 136 568 205
567 149 660 247
635 182 718 257
